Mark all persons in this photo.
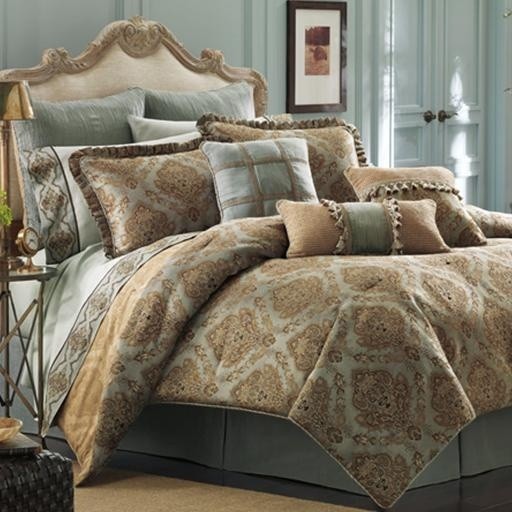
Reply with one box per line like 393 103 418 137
308 45 328 74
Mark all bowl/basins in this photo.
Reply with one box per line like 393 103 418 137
0 417 24 444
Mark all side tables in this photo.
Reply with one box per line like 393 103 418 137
0 264 61 451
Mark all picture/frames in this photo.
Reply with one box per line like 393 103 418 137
286 0 348 113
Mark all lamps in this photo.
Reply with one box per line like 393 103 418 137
0 78 36 269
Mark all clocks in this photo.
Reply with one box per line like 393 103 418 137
14 226 44 274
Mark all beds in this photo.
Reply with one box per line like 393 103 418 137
1 18 512 496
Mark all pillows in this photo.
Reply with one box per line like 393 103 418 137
199 136 320 222
196 112 370 204
29 86 147 147
343 165 486 248
134 83 259 122
128 115 197 144
11 117 127 265
68 139 222 261
276 198 452 259
